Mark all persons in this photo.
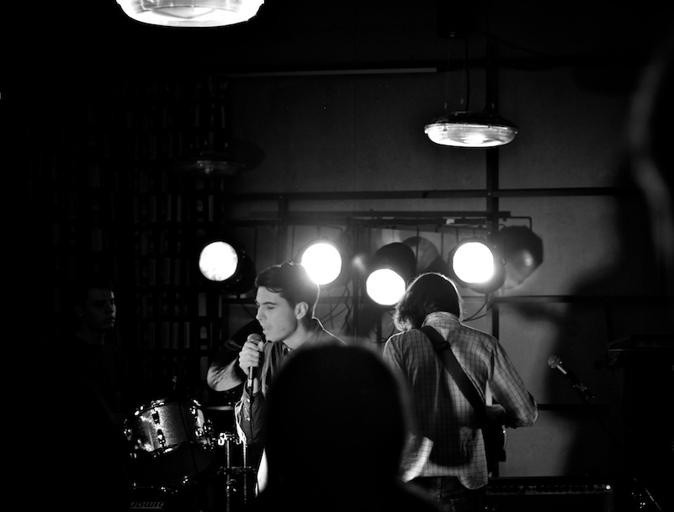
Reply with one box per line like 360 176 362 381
35 280 174 412
376 270 538 511
243 339 448 511
201 258 345 491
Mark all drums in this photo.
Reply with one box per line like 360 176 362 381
217 466 258 512
124 400 214 482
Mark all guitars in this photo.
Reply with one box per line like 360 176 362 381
485 422 507 472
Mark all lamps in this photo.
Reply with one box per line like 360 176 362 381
423 37 521 150
115 0 265 29
198 237 256 296
360 239 506 309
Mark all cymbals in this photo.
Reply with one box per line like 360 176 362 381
216 407 236 411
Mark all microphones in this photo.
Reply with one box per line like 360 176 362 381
248 334 263 396
545 352 588 392
